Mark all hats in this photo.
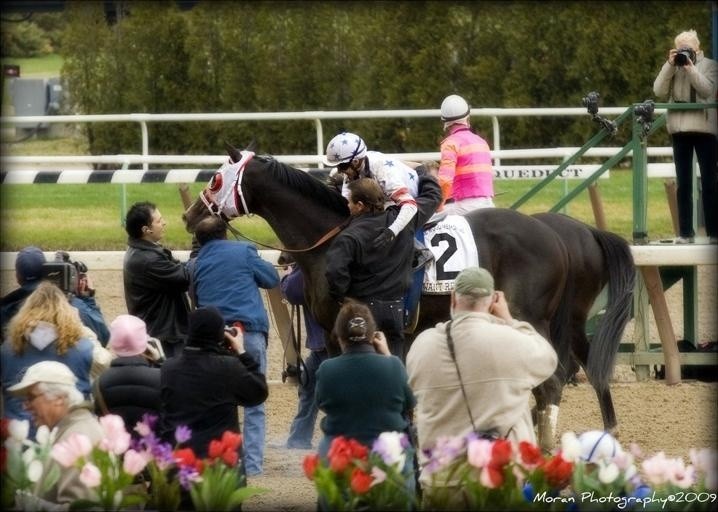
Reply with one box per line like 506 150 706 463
107 314 148 356
452 266 495 298
5 358 77 399
14 244 46 290
185 306 226 345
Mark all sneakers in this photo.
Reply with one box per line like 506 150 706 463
674 236 690 245
709 236 718 245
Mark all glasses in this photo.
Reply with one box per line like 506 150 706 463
337 163 351 171
21 392 47 402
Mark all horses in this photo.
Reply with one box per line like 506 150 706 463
182 140 570 457
278 174 636 438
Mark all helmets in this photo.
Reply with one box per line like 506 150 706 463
320 132 368 169
439 94 471 120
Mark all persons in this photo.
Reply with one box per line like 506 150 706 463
404 267 559 511
326 160 443 362
122 201 190 359
421 95 497 226
159 303 268 512
0 281 115 455
189 216 281 478
326 132 434 272
653 29 718 245
0 246 111 347
5 360 117 512
267 265 336 449
314 296 418 511
89 312 168 439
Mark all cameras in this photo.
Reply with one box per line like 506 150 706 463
635 101 655 128
582 91 601 117
671 49 696 67
222 323 237 349
144 336 156 358
479 430 502 443
